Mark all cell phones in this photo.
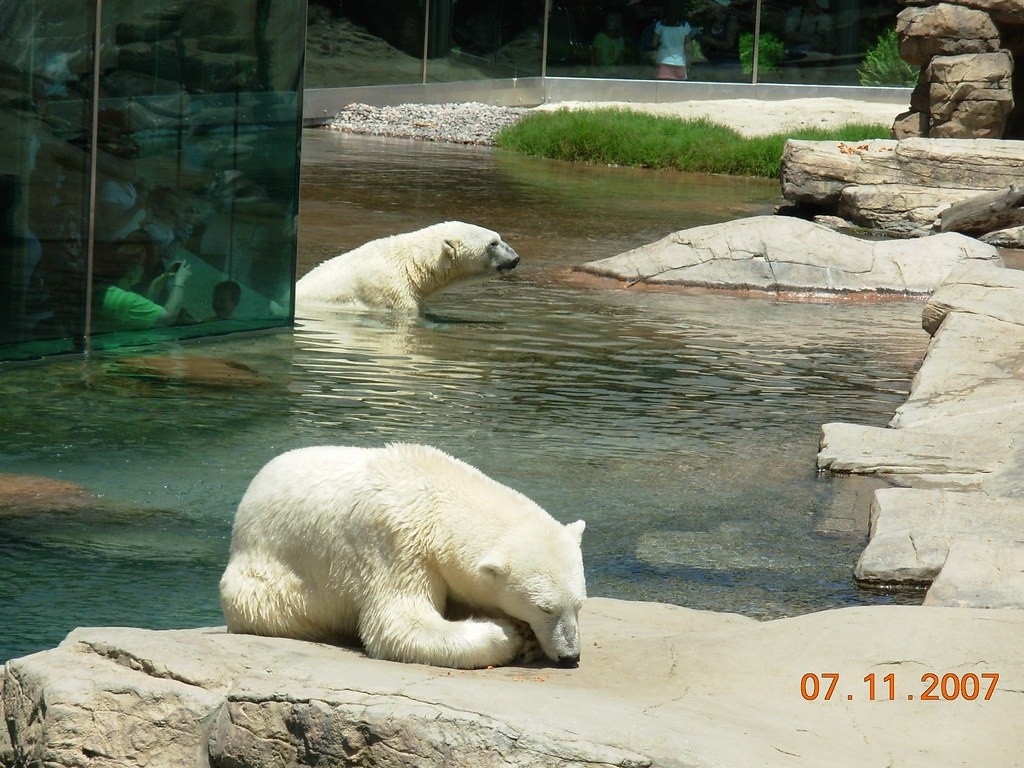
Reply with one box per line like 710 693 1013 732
167 261 184 276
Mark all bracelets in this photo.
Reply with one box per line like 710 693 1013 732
172 281 186 288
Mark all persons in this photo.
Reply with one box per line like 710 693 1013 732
588 0 873 82
1 33 244 335
